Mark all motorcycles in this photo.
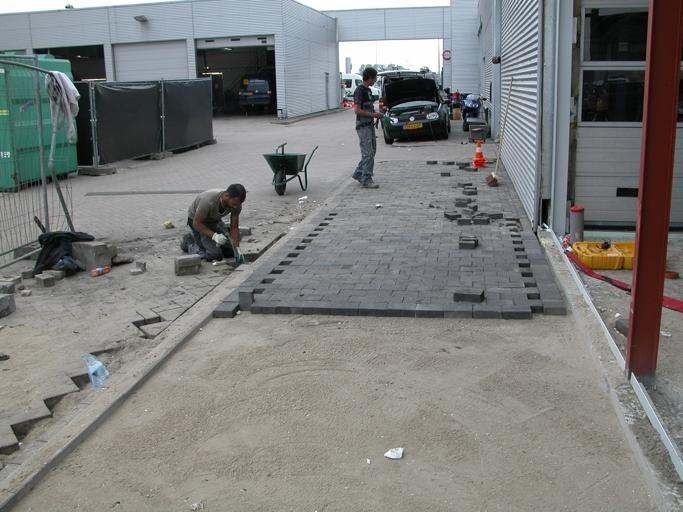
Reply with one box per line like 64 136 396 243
460 94 487 120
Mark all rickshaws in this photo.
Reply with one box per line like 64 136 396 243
262 142 319 196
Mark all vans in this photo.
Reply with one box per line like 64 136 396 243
342 73 381 102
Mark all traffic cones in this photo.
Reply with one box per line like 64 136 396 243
473 139 485 167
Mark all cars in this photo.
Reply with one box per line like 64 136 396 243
237 78 272 113
380 79 451 144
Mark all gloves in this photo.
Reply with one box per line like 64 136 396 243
234 246 245 263
211 231 227 246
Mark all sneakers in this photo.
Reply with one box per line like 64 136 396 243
362 176 380 189
181 233 193 252
352 169 366 183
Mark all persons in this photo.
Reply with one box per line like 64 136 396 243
349 66 384 189
180 183 247 262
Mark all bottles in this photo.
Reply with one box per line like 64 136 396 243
475 140 482 158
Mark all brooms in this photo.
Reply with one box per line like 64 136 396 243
485 76 513 187
